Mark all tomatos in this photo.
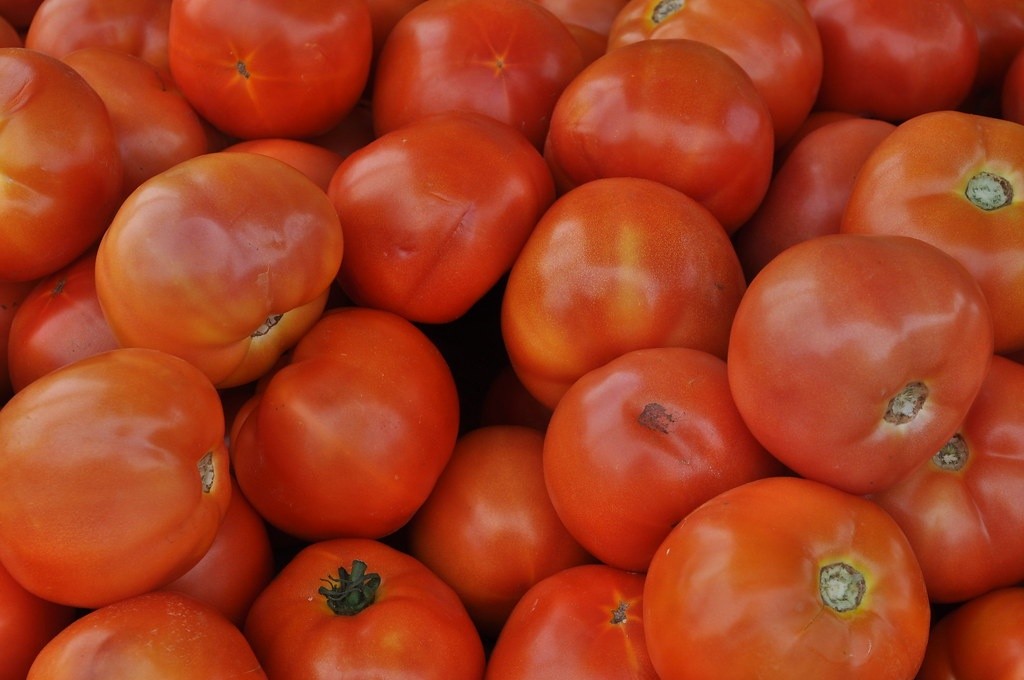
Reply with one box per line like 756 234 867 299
0 0 1024 680
